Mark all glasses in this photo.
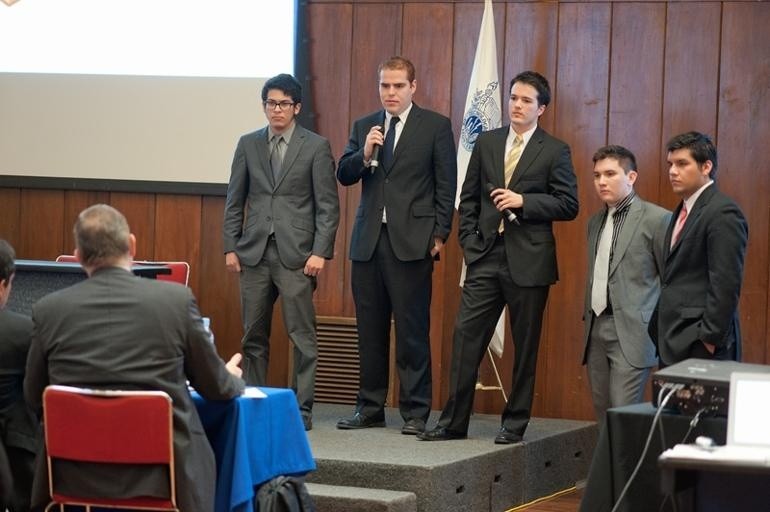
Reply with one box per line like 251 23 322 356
265 100 296 111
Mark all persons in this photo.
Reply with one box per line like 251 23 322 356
0 239 35 512
223 74 340 433
336 57 458 436
579 147 675 511
421 71 579 445
659 131 748 450
23 204 244 511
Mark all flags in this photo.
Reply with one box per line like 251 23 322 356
454 2 506 358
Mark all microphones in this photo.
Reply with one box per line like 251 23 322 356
367 128 384 173
484 182 522 226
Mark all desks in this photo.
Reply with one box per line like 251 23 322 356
657 443 769 479
3 258 173 319
188 386 316 512
576 400 727 512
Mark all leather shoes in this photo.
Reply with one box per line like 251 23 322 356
494 426 524 444
416 425 468 440
336 411 387 429
302 416 313 431
401 419 426 434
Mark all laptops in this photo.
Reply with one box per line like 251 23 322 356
659 372 769 467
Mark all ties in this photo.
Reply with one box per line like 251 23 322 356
590 206 617 318
381 116 400 173
497 133 525 234
267 135 284 236
670 207 688 253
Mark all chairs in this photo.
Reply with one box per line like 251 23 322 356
42 384 181 512
53 254 190 290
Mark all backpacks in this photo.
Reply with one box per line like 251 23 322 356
256 475 319 512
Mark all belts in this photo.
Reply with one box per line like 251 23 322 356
269 233 276 241
592 304 616 315
